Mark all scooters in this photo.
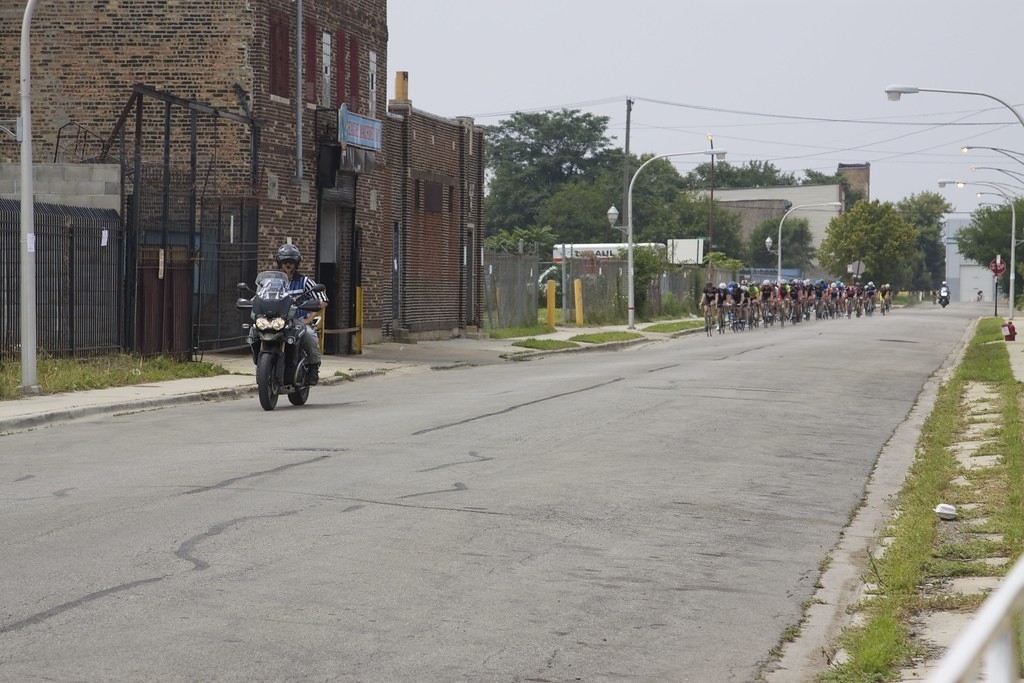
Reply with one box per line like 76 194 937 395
939 292 950 308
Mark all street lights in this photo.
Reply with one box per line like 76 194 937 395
777 202 842 280
938 179 1015 321
627 149 728 329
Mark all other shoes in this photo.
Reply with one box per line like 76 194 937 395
305 364 318 386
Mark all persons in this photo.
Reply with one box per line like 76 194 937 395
938 280 951 304
249 244 329 385
699 279 892 332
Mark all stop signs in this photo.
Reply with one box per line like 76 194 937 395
989 258 1006 276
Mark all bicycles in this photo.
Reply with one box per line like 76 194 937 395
977 294 986 303
701 294 892 337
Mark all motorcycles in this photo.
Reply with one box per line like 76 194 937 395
235 271 326 411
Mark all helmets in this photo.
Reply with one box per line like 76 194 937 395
719 282 726 289
728 279 891 292
276 244 301 262
942 281 947 284
707 283 712 287
